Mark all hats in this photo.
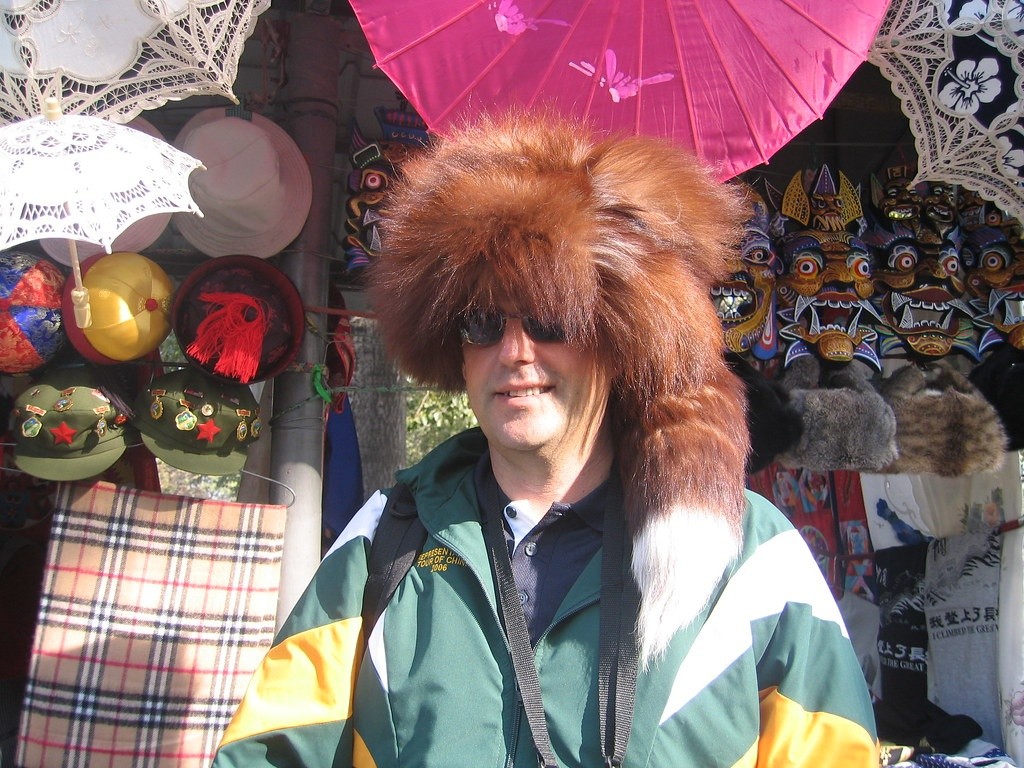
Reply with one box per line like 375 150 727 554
39 110 173 268
62 252 178 366
879 358 1008 477
775 354 900 471
12 368 137 481
128 367 263 475
0 250 67 374
172 256 305 387
967 343 1024 452
724 353 805 475
172 105 313 259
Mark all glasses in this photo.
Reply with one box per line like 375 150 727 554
454 305 565 344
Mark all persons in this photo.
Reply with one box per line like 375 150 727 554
200 113 884 768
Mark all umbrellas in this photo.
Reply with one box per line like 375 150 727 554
1 0 1024 222
0 97 208 330
